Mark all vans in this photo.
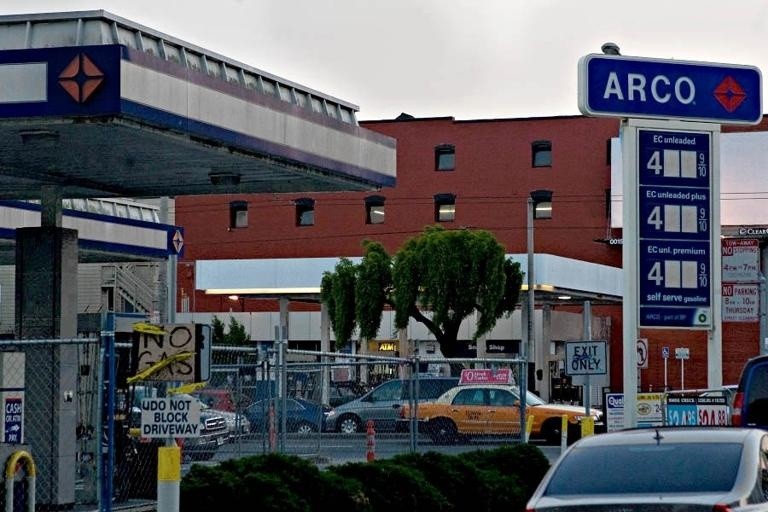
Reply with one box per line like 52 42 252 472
323 376 462 436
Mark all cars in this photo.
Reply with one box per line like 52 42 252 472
397 383 603 447
118 372 332 467
522 351 767 512
